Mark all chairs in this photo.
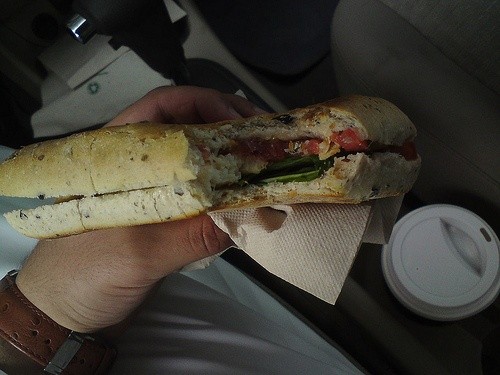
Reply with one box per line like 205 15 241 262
328 2 499 245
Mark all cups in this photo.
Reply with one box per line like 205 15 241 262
381 205 500 320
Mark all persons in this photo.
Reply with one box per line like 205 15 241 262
0 85 318 375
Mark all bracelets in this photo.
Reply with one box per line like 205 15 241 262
0 269 116 375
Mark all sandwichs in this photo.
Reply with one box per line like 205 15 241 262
0 94 422 237
